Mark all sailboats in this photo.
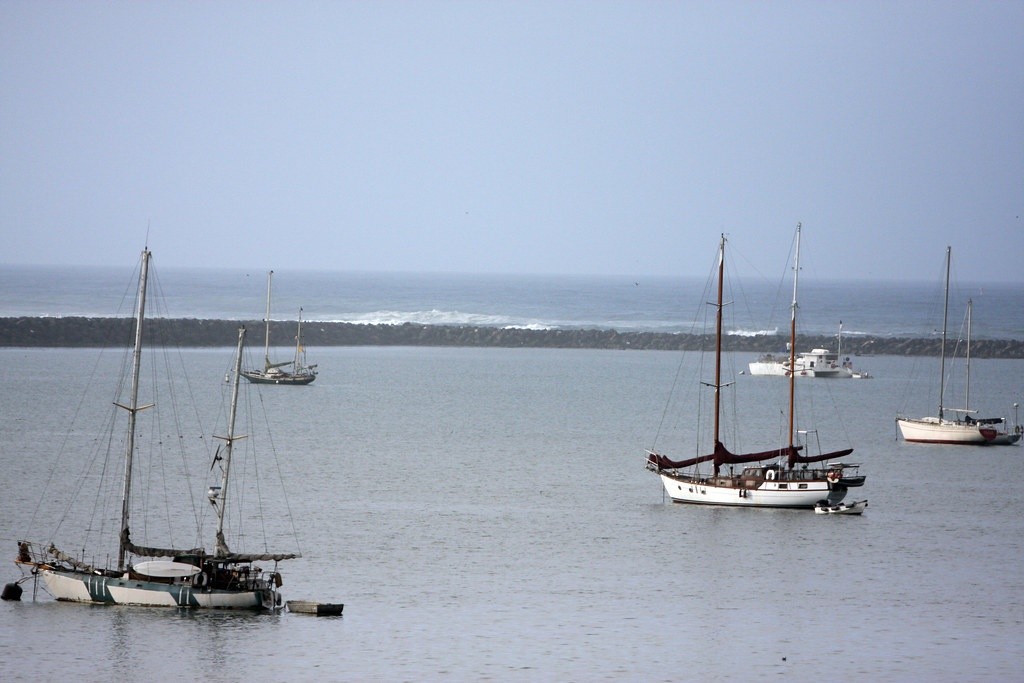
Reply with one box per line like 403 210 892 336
641 220 872 517
892 242 1023 449
228 270 319 386
748 220 869 380
0 223 344 617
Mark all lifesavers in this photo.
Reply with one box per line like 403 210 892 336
1015 426 1019 432
193 571 208 585
766 469 775 480
